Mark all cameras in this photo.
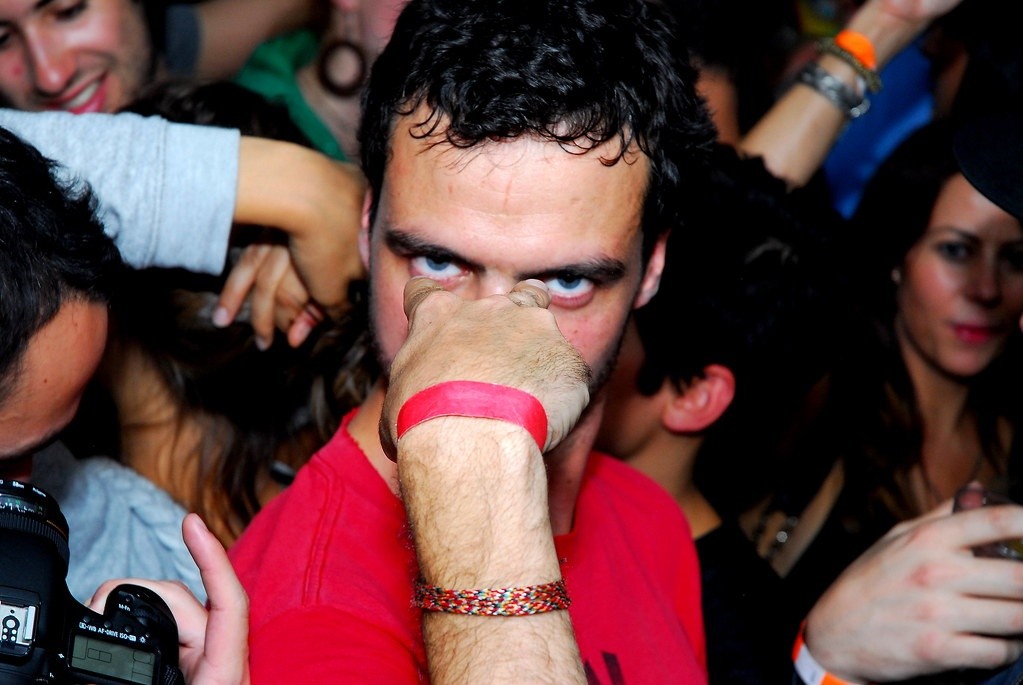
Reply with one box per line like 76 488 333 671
0 477 183 685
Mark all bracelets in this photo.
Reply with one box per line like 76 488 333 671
792 619 857 685
816 35 880 95
413 580 571 616
397 381 548 453
797 60 866 121
834 29 876 72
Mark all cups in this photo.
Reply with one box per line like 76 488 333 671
951 490 1022 561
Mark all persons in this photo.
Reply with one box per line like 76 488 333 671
0 0 1023 685
